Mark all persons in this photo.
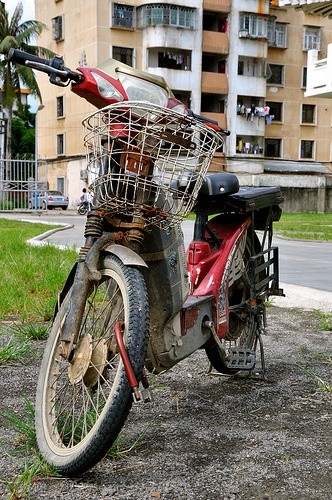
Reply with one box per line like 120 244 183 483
77 188 87 206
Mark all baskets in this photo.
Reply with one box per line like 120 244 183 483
82 101 224 230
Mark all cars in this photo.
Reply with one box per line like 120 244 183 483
28 190 69 210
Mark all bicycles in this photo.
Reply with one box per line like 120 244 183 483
77 201 88 215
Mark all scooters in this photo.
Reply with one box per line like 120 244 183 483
4 45 285 481
